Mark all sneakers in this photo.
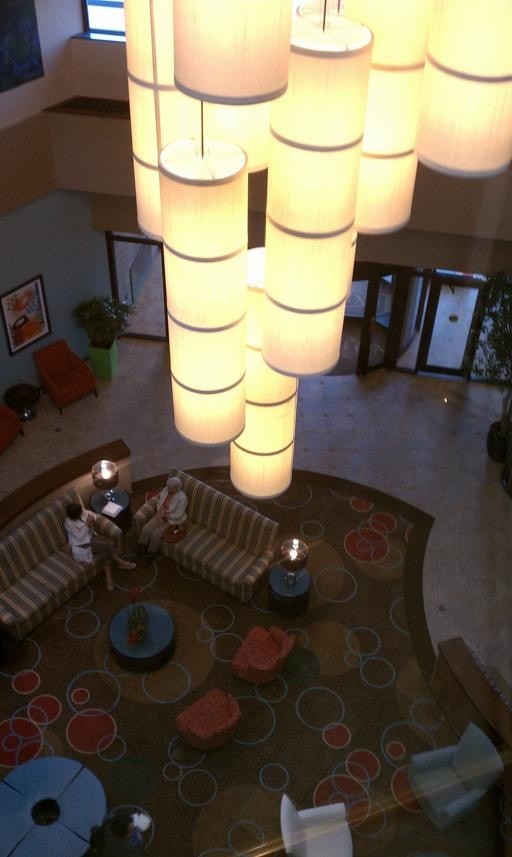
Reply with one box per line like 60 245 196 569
136 543 157 561
106 576 115 591
118 561 137 571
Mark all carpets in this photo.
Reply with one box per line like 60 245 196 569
0 466 512 857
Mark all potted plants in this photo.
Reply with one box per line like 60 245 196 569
471 269 512 462
71 297 138 381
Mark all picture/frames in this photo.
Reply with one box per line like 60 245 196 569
0 273 53 357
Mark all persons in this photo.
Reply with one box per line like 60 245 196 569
100 813 146 856
127 477 187 566
62 501 138 593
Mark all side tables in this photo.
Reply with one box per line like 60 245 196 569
267 566 312 620
91 490 131 532
4 385 40 422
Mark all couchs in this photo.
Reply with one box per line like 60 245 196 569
134 464 278 603
0 487 124 645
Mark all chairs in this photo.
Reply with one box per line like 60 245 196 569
33 340 98 415
230 624 297 684
280 791 354 857
174 688 242 752
409 722 506 832
0 404 24 459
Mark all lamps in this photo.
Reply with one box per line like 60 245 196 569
120 0 512 499
92 459 120 500
281 538 310 586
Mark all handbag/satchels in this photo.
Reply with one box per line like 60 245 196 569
71 545 93 563
163 524 185 543
